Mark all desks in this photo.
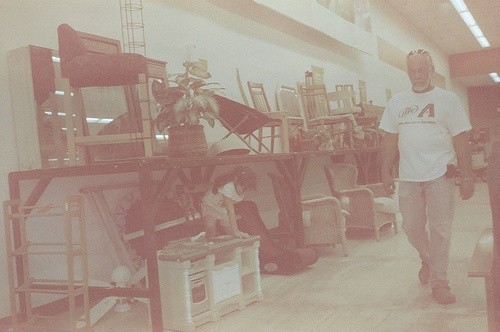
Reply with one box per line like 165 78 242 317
145 235 264 331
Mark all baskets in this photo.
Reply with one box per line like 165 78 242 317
167 123 209 158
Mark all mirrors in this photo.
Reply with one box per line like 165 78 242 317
48 51 145 166
30 45 169 167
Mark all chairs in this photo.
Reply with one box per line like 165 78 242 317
234 201 318 274
324 163 398 242
207 93 271 154
57 23 152 167
248 71 385 154
269 172 351 257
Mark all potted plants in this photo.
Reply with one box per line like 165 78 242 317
152 59 226 157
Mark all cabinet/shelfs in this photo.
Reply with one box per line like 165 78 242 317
3 196 90 332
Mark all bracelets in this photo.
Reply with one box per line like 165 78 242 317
462 178 473 181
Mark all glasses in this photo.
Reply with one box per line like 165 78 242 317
406 49 431 59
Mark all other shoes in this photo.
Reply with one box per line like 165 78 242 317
418 262 430 283
432 285 456 304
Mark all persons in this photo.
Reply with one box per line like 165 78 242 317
378 50 475 304
200 165 257 239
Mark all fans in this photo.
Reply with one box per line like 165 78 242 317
74 266 147 329
